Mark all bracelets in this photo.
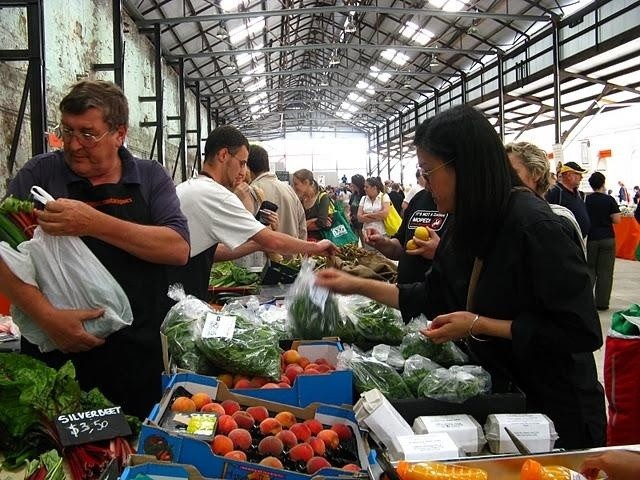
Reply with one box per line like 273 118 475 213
468 314 487 343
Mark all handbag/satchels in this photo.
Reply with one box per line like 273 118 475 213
316 191 359 249
381 193 403 238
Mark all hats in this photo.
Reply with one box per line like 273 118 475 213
560 161 588 175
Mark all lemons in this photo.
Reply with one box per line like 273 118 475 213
415 226 429 242
406 239 419 250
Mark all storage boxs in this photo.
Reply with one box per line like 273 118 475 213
119 337 368 480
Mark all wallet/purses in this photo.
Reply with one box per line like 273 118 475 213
255 201 279 227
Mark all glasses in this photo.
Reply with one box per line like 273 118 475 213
216 149 247 169
415 159 456 187
355 174 361 180
57 122 111 148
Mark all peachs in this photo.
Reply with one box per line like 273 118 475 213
169 391 363 473
218 350 339 392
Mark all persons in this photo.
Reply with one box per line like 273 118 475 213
578 448 640 479
0 77 192 423
176 125 428 316
314 103 610 452
363 166 448 325
504 141 640 311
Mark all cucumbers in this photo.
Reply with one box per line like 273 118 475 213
0 214 28 243
0 226 21 253
